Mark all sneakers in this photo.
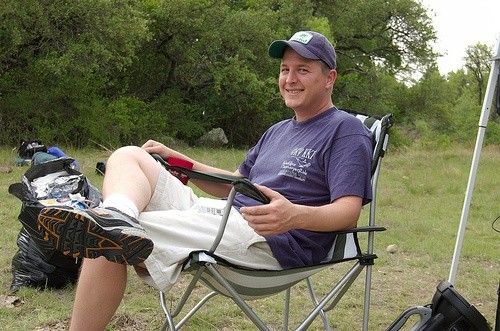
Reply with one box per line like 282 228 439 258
37 205 154 266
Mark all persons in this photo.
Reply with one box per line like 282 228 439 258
36 30 376 331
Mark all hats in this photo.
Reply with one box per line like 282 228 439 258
267 30 336 70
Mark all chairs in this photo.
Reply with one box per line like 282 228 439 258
160 109 394 331
